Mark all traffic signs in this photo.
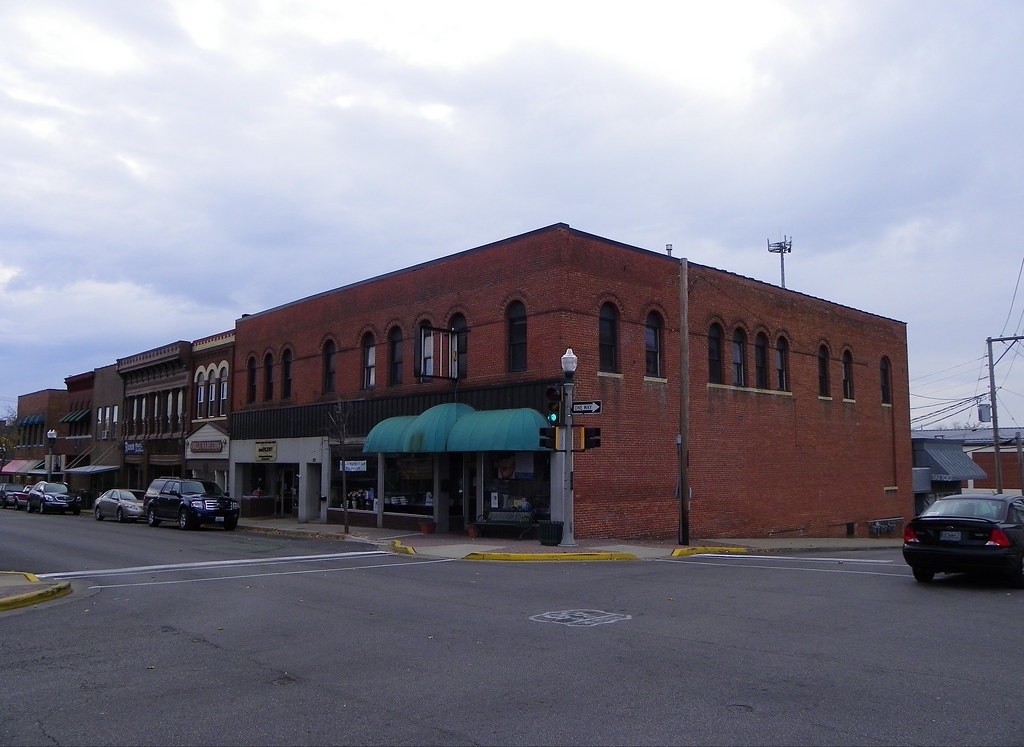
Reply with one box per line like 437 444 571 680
572 399 603 414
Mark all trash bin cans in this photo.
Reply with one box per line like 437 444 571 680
537 519 565 547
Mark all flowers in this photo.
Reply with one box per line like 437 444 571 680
348 489 363 500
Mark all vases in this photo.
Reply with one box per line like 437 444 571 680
502 494 509 509
352 500 357 509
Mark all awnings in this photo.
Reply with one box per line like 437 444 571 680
922 448 988 482
13 414 44 425
61 464 120 475
0 459 46 475
58 409 91 423
360 402 556 452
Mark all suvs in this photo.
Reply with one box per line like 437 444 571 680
143 476 241 531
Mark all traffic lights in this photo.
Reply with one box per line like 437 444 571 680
538 425 560 451
544 385 562 428
578 427 602 451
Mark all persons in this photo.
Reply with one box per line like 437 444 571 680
497 451 515 479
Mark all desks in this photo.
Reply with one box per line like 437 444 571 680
383 503 433 515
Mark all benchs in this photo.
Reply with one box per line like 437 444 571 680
473 511 536 540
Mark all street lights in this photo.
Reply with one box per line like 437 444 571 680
46 429 57 482
559 349 580 545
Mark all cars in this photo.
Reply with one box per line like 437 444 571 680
27 481 82 515
0 483 26 508
902 493 1024 593
11 484 34 510
94 489 148 523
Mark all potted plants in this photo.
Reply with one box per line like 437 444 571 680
418 515 434 533
464 522 479 537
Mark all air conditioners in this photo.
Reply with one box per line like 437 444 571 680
102 430 109 439
